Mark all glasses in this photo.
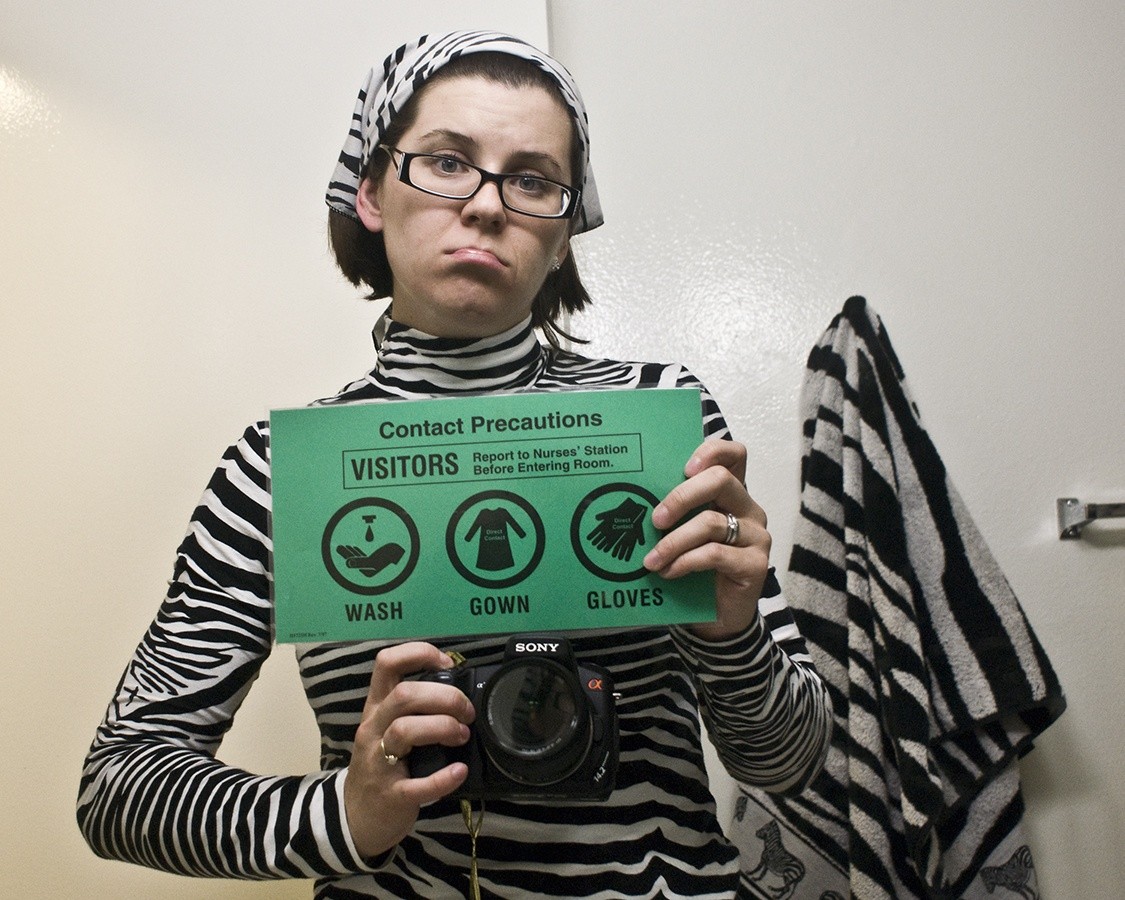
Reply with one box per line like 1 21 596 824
376 143 581 220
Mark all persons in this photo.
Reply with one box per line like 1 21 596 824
76 30 836 900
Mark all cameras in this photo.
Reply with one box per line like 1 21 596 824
397 635 620 804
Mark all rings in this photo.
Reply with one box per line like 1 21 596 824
724 512 738 545
381 738 405 766
